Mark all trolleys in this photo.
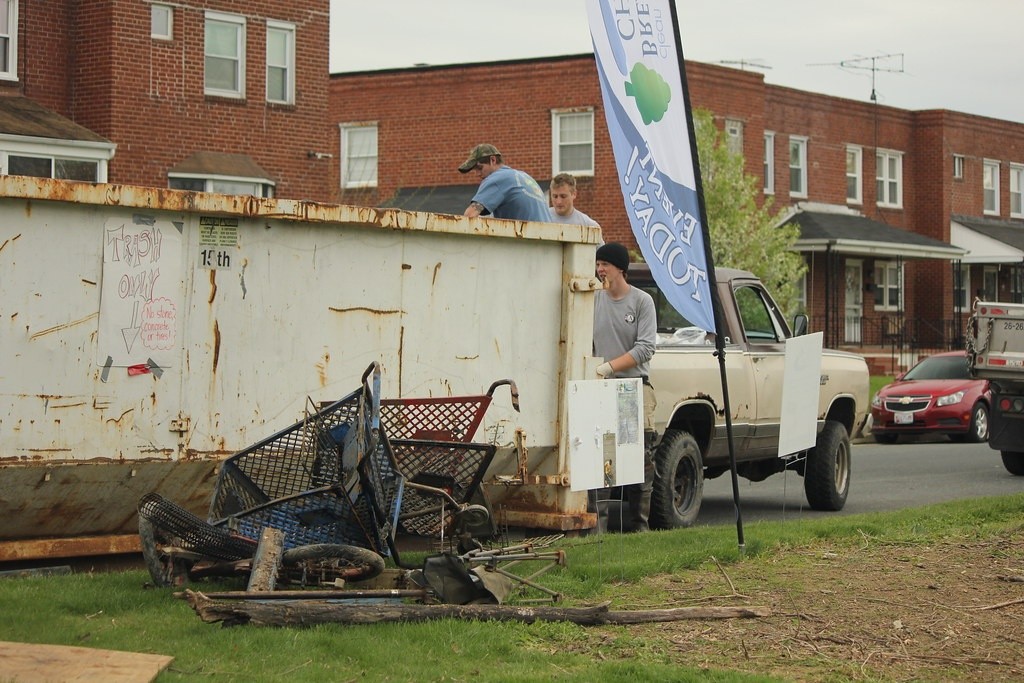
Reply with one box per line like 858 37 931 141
203 363 523 563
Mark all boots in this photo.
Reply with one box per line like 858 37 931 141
585 489 610 534
627 492 651 532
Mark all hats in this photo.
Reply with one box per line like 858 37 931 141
457 144 501 174
597 241 630 272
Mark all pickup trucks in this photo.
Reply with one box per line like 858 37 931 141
622 263 870 527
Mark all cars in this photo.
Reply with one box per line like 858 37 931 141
869 349 994 443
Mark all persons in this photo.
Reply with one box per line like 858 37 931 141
593 243 657 532
457 144 553 222
547 174 605 250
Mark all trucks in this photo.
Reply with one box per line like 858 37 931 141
966 296 1024 475
